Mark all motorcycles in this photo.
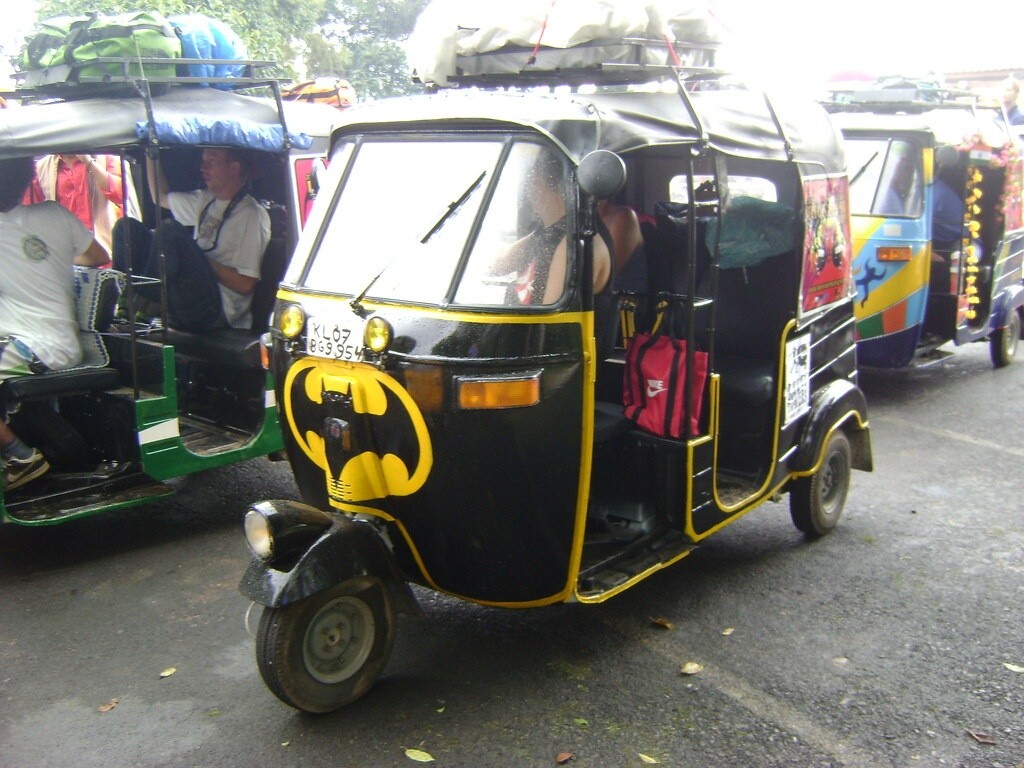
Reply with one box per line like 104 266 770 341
1 79 353 534
244 27 874 717
822 78 1024 373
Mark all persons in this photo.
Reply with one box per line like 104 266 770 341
996 82 1024 140
885 157 970 264
21 153 129 267
111 144 274 329
1 156 110 491
487 145 645 365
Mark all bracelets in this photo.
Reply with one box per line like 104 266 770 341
87 160 93 174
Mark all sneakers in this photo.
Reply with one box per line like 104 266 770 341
2 446 50 493
104 306 165 335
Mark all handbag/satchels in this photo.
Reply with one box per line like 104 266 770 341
623 301 709 439
